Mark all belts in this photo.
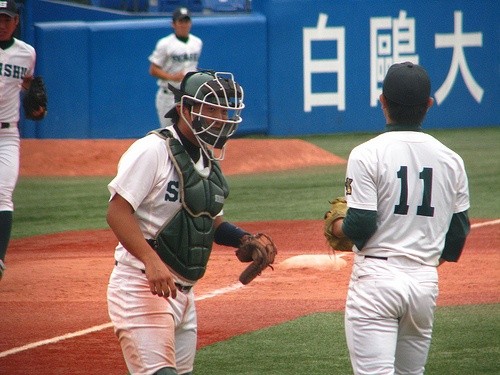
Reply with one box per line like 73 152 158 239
365 254 388 260
115 262 192 293
1 122 10 129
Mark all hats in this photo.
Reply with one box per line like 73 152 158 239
0 0 17 17
381 62 429 106
173 8 190 20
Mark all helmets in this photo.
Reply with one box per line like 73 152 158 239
165 72 230 118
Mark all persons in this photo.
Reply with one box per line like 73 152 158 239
105 69 277 375
0 0 47 282
148 8 203 128
324 61 470 375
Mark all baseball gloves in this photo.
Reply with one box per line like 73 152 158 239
236 232 277 285
323 196 354 252
23 77 47 120
